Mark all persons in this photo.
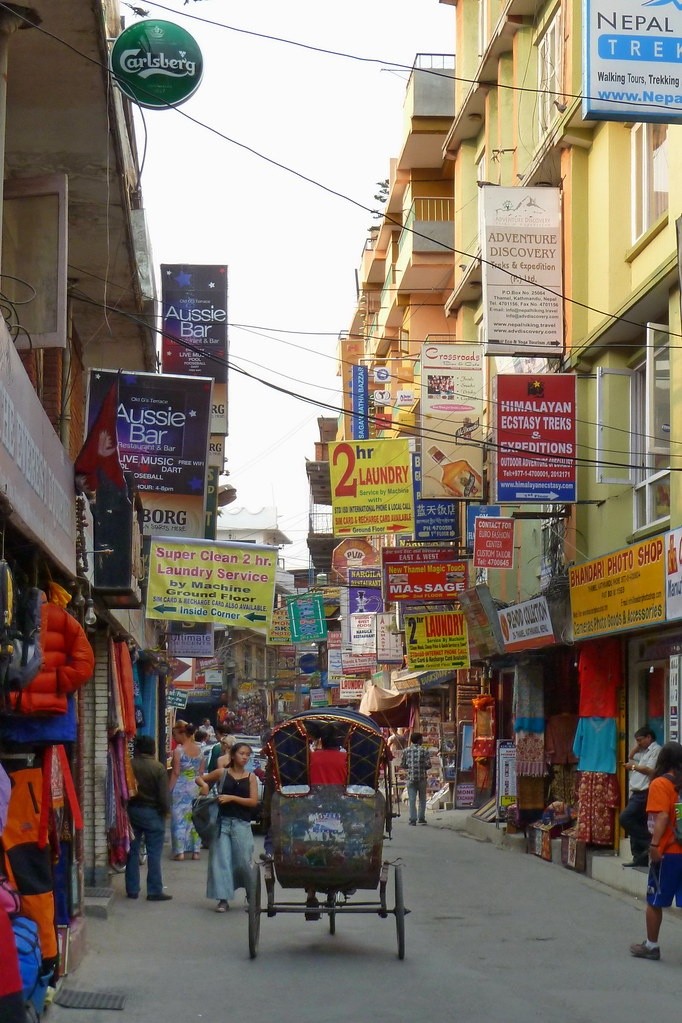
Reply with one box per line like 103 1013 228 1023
387 727 406 751
126 736 173 901
168 718 272 861
305 736 356 921
400 732 432 827
196 742 258 913
629 741 682 961
618 726 663 867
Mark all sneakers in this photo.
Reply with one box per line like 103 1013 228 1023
630 940 660 960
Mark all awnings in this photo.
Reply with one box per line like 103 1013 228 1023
359 684 407 716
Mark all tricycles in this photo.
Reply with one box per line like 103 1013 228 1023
243 706 412 960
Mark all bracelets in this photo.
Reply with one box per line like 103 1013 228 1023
649 843 659 847
632 764 636 773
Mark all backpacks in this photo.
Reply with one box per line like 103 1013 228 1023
662 773 682 847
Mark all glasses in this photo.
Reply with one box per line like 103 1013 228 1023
637 737 646 742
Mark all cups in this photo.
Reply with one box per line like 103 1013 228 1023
674 803 682 821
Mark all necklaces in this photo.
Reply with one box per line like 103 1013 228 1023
231 768 244 785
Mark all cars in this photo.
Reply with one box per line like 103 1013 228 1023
200 739 268 823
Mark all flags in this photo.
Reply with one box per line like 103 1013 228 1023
73 368 126 491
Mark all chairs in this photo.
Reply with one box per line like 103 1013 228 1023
309 748 348 791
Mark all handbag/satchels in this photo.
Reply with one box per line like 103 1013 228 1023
190 796 223 840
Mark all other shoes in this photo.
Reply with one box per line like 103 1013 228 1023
176 854 185 860
305 897 320 921
621 855 653 867
192 853 200 860
216 902 229 912
409 822 417 826
632 833 653 853
147 892 172 900
347 889 357 895
418 819 427 823
127 893 138 899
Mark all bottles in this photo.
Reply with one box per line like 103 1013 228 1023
429 449 482 497
